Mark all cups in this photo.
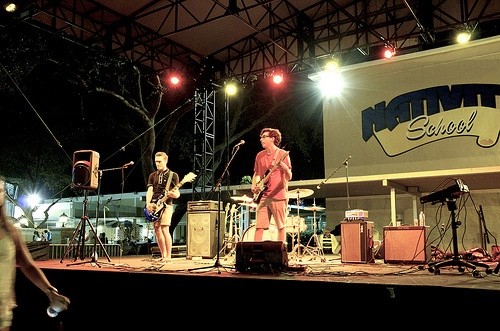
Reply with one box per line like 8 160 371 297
397 221 401 226
414 219 418 226
47 297 65 317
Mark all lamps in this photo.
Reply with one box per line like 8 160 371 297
385 44 398 59
456 23 476 44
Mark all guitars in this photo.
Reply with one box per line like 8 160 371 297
143 171 197 222
252 150 290 204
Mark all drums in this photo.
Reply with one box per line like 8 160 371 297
241 214 306 243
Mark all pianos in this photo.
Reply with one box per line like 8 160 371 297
419 182 494 278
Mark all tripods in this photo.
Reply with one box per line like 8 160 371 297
187 147 240 275
57 190 112 262
219 193 327 262
66 165 128 268
428 199 493 276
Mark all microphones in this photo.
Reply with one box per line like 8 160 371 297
234 139 246 148
123 160 135 167
317 181 325 190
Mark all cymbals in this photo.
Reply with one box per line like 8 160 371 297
288 188 315 199
230 196 253 202
238 202 258 207
303 206 327 211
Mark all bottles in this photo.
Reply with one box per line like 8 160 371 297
419 211 425 226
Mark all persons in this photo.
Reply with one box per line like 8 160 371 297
146 151 180 263
98 232 105 244
0 176 70 331
85 230 95 244
42 230 49 241
251 128 292 242
33 231 41 241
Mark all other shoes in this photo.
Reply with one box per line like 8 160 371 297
158 258 172 263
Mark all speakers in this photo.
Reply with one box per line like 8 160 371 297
339 221 373 264
185 209 226 259
382 226 432 264
71 149 100 190
234 241 288 274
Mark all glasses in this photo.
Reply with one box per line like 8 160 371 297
156 160 165 163
261 135 273 138
